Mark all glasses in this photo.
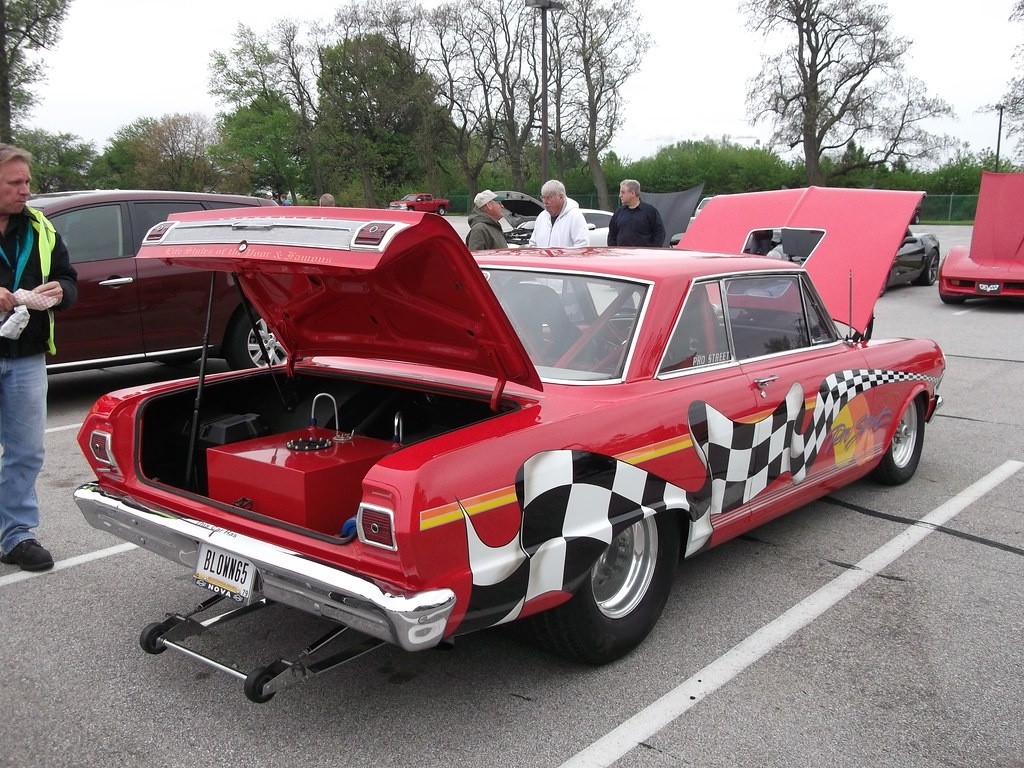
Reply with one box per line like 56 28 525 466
540 195 562 200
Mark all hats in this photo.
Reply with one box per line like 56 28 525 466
473 189 507 209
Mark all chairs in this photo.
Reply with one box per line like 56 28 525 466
515 281 563 357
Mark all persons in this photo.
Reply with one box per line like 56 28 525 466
319 193 335 207
466 189 509 249
529 179 590 294
280 194 292 206
0 142 78 569
750 229 773 255
607 179 667 307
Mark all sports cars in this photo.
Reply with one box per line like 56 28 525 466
772 224 941 296
937 168 1024 307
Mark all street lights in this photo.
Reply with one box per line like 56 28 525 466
523 0 562 182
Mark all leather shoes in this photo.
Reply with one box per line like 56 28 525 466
1 539 53 570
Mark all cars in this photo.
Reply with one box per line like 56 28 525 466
491 190 615 248
68 184 952 687
26 190 286 377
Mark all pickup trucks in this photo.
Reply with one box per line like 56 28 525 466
387 193 450 217
